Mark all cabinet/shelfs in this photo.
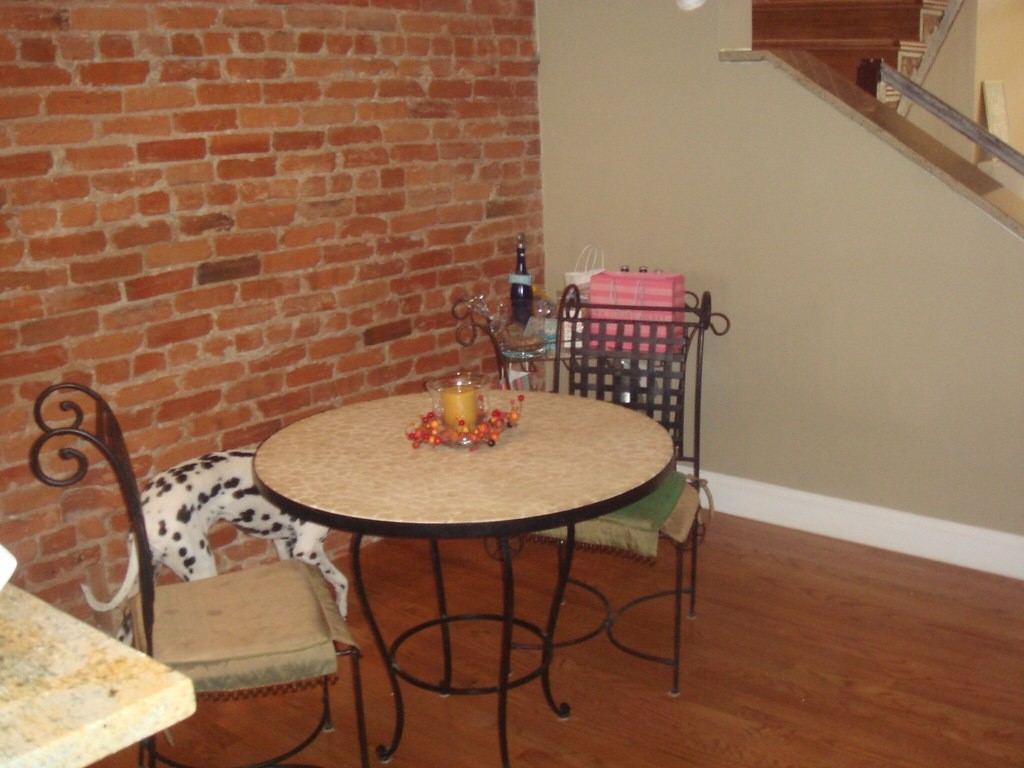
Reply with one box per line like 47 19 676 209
452 291 730 561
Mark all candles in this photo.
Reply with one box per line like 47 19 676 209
441 387 478 432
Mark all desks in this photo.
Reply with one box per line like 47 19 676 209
254 387 677 768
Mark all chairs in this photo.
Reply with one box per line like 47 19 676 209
495 284 730 698
29 383 372 768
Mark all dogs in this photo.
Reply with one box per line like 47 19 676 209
80 441 348 647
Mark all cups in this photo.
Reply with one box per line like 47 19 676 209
427 372 489 450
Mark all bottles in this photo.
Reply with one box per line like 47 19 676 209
508 232 534 325
620 265 663 275
855 57 884 98
